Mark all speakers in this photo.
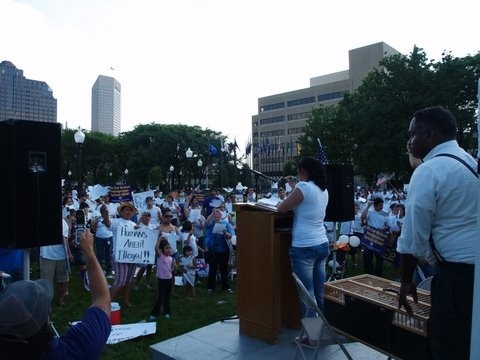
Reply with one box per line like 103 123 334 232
324 164 355 222
0 118 63 249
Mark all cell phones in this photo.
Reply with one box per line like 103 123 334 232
76 210 86 244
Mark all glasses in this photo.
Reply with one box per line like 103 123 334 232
165 215 173 218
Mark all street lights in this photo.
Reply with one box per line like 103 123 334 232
185 148 193 195
197 159 203 190
68 171 71 190
125 169 129 186
170 166 175 192
74 126 85 193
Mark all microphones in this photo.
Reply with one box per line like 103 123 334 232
280 177 297 183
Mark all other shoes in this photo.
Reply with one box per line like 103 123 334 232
222 286 233 293
294 335 319 350
207 288 214 294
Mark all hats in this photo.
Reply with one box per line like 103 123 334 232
117 201 136 218
2 278 55 340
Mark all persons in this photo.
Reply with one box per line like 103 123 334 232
0 158 421 360
397 106 480 360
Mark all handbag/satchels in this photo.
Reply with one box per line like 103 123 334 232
204 249 218 264
225 232 236 266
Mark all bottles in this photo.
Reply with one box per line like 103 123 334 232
236 182 244 203
271 183 279 199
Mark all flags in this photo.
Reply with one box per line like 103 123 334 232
375 174 387 186
209 135 332 162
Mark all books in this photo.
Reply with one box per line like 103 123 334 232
254 198 279 213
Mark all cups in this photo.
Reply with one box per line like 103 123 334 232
110 302 121 325
